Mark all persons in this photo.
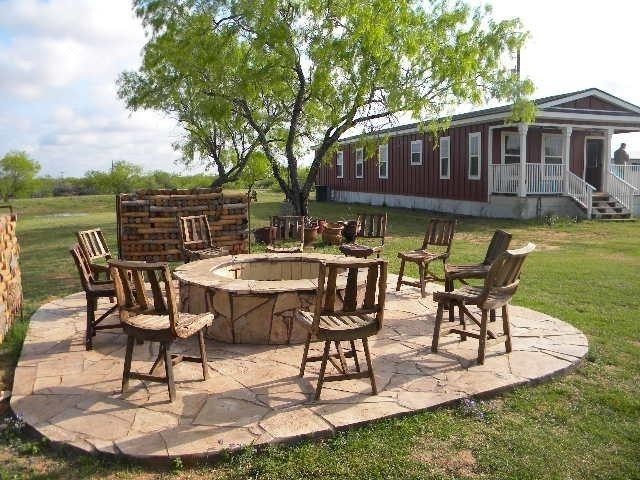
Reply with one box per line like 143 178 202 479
614 142 629 179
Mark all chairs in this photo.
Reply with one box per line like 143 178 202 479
69 243 147 351
103 258 215 399
294 258 390 397
173 213 225 264
395 216 457 299
337 213 389 258
438 228 514 324
74 225 144 288
428 241 536 367
263 213 308 253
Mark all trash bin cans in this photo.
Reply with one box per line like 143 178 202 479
315 185 327 201
